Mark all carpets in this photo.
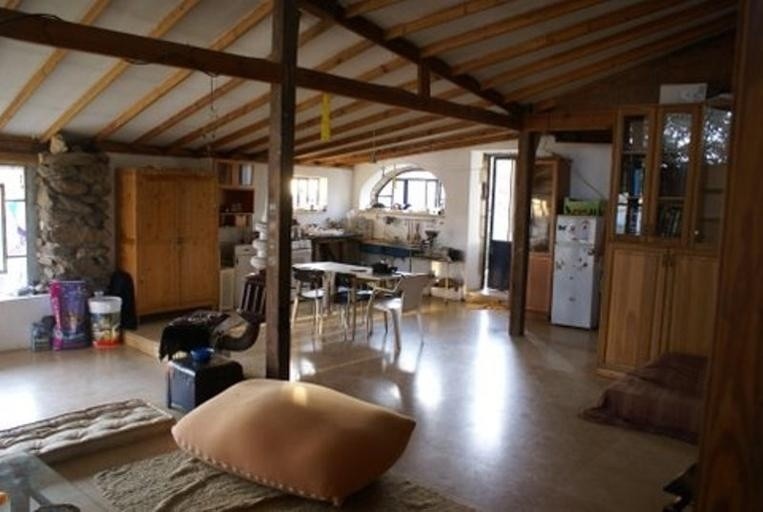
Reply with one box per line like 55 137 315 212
90 453 477 511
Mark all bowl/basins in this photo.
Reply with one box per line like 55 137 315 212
190 347 215 362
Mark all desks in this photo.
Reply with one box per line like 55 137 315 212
291 260 373 323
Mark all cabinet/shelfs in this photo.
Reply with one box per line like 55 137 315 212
220 186 254 311
114 167 221 326
597 101 737 381
359 246 430 296
526 158 571 312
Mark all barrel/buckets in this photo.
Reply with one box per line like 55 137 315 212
89 296 123 346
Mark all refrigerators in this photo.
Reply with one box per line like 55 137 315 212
549 214 607 331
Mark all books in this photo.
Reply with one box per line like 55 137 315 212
621 117 688 240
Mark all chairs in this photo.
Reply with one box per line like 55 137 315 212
290 273 430 353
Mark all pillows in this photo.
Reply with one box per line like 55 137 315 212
170 378 417 506
